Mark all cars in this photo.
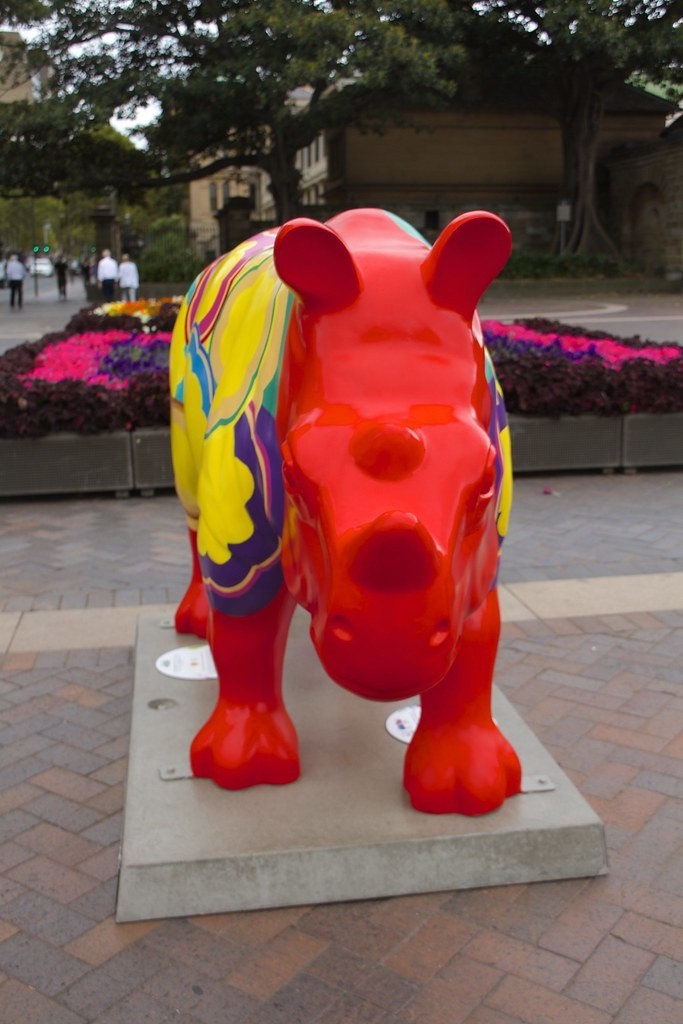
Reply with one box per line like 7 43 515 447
30 257 53 276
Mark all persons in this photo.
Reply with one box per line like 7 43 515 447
96 249 140 303
6 254 26 310
54 255 68 300
81 259 91 282
